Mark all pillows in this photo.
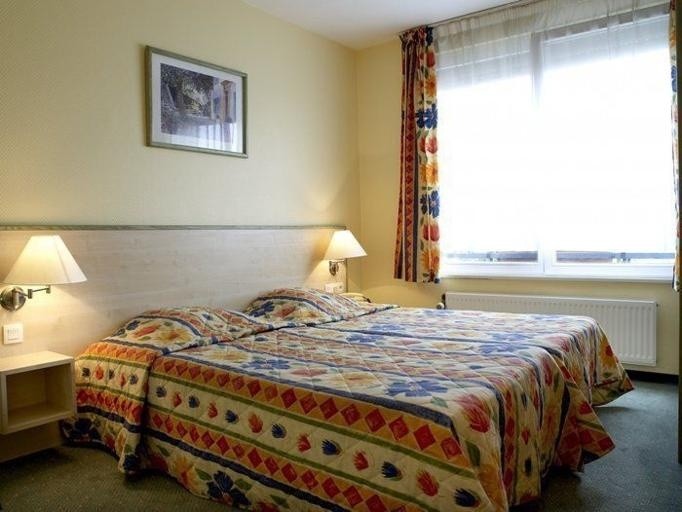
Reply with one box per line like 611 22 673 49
247 288 355 324
119 306 237 346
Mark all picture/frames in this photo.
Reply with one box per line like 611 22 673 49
145 46 249 158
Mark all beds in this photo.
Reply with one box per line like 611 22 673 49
0 225 633 511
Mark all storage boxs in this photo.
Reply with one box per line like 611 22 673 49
435 292 658 368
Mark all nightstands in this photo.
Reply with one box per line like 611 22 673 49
0 351 78 467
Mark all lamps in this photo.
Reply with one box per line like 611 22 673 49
0 235 88 312
322 230 367 276
